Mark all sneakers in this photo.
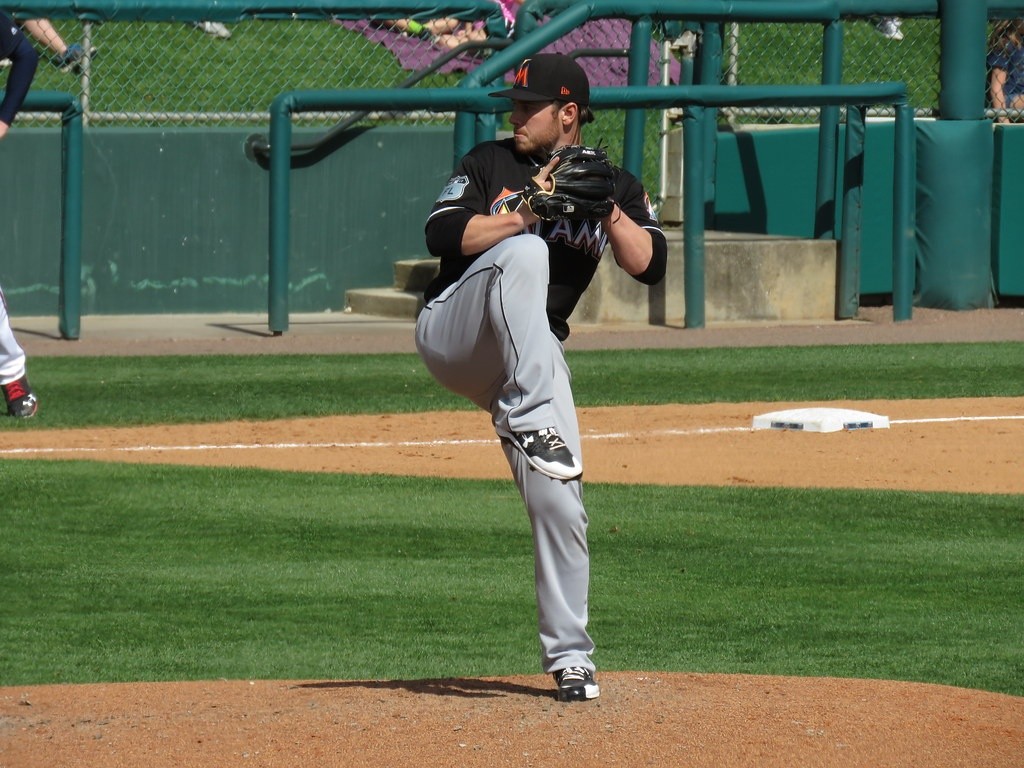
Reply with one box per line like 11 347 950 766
1 375 38 417
550 667 600 701
495 421 583 484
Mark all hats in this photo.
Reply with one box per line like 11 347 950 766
488 51 590 108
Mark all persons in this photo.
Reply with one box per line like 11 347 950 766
986 19 1024 123
415 52 667 702
17 20 98 72
372 0 525 50
0 11 39 418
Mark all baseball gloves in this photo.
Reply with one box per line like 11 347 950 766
528 145 616 221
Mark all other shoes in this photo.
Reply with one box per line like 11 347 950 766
205 22 230 38
55 45 99 76
